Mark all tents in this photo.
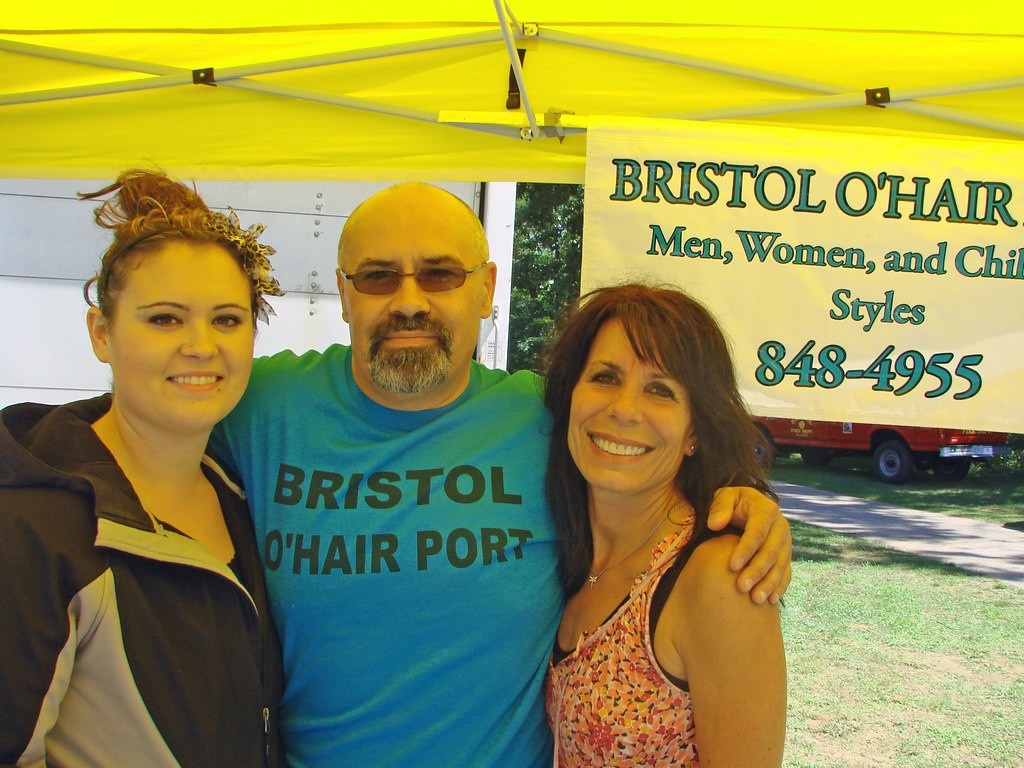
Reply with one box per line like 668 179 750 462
0 0 1024 180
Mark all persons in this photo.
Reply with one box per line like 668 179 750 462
0 168 286 768
211 183 791 768
533 276 787 768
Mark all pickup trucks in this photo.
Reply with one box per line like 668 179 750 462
749 414 1014 483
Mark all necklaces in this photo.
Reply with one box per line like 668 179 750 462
588 500 689 589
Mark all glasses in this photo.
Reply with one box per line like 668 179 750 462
339 262 487 296
